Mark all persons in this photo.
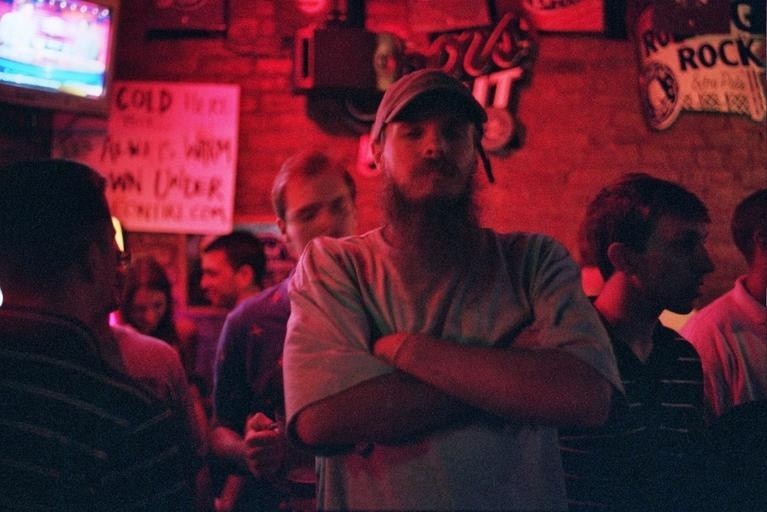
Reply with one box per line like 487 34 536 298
1 158 206 512
116 254 198 377
182 227 265 512
559 174 718 511
100 316 206 447
678 187 766 412
205 148 363 512
279 65 617 511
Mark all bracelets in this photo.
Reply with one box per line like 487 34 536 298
390 330 412 368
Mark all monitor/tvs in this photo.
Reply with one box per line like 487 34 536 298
0 0 123 120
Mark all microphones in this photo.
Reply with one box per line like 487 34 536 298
477 144 495 183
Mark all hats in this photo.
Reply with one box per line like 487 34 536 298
372 68 487 142
